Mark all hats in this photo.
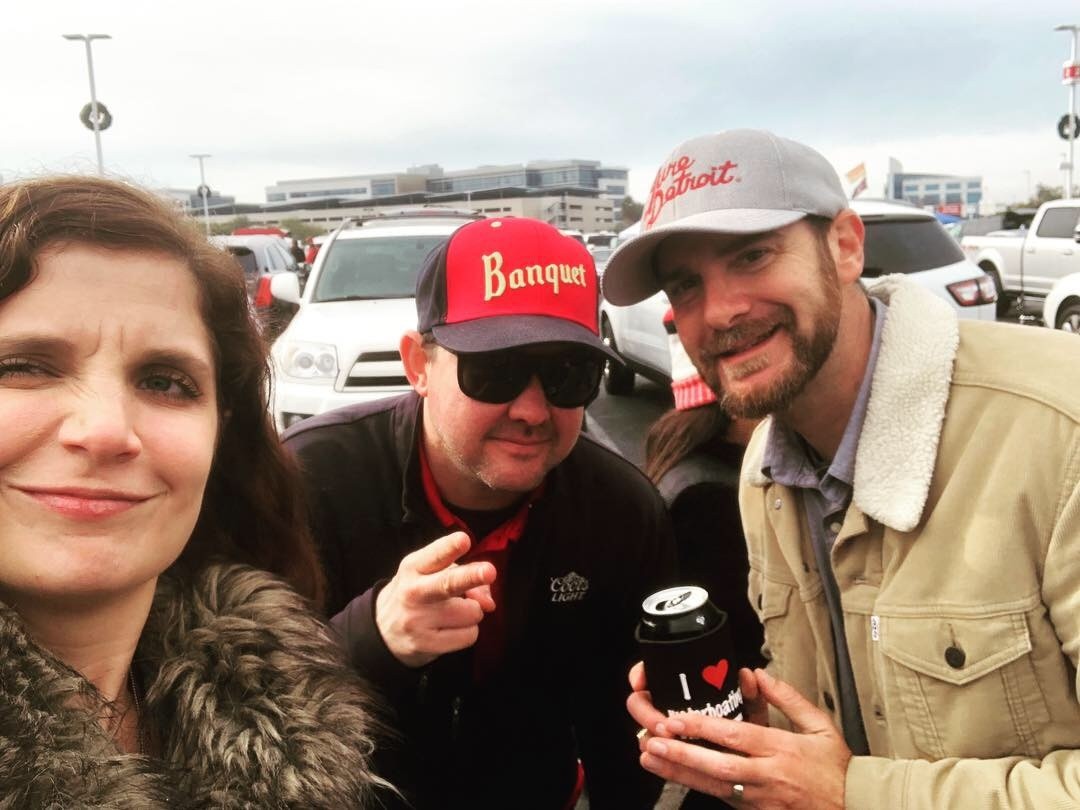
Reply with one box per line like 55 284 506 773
602 131 848 306
415 216 628 364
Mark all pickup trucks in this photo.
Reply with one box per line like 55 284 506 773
958 197 1080 335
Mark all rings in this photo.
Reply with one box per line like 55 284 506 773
729 783 744 801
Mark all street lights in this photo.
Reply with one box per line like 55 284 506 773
1052 24 1078 200
189 153 212 235
62 32 113 177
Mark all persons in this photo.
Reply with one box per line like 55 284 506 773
290 238 317 267
0 177 412 810
279 217 689 810
602 129 1080 810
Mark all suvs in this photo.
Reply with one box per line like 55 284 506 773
599 195 1000 397
259 207 490 441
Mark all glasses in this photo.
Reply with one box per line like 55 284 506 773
455 342 606 409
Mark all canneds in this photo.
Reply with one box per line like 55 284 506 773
638 585 721 640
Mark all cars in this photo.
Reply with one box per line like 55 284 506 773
560 229 620 276
204 233 297 292
